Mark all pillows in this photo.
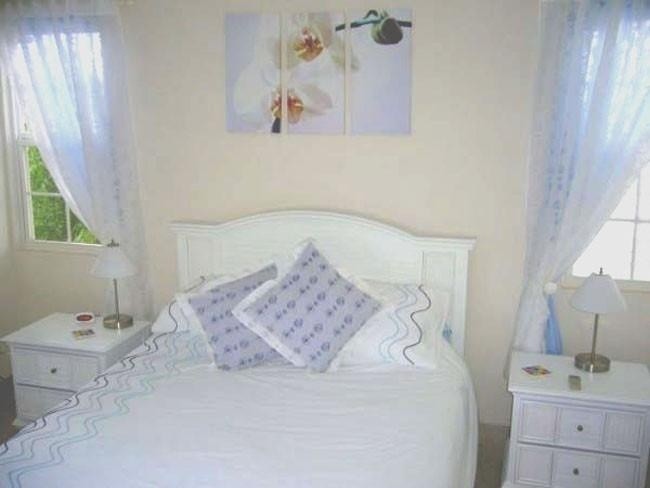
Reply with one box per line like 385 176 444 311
173 260 295 372
330 273 452 372
232 237 384 373
153 270 247 333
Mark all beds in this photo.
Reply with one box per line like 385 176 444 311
0 208 478 488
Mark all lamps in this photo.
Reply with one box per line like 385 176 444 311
90 237 141 329
569 268 626 372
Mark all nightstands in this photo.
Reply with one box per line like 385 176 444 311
1 311 151 427
501 352 650 488
